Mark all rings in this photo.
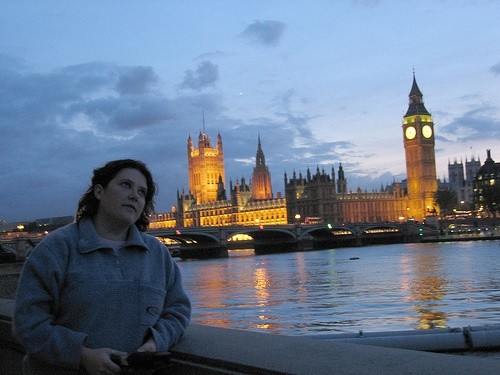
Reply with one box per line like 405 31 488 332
111 349 116 356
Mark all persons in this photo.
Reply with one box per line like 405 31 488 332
12 159 191 375
